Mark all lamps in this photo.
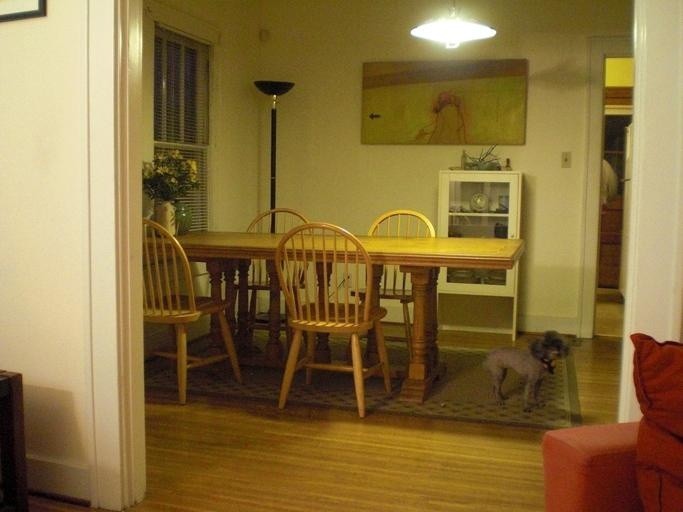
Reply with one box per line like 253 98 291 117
410 0 498 50
254 80 295 232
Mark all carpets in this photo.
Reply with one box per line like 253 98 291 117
143 333 584 432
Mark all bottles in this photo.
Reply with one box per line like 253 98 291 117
175 205 192 234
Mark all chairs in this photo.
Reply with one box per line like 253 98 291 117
141 216 242 405
274 222 392 418
347 209 436 361
542 419 640 512
229 208 313 351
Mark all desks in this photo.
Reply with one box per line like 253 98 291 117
140 229 528 408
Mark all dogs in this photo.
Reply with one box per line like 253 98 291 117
482 330 570 412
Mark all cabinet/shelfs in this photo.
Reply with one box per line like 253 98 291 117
434 170 523 341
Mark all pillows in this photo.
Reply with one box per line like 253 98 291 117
631 331 683 511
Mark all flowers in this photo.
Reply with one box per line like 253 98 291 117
142 149 197 202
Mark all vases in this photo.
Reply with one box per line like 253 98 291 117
156 202 176 236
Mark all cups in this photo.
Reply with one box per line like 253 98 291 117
449 216 464 237
495 224 507 237
499 196 509 210
